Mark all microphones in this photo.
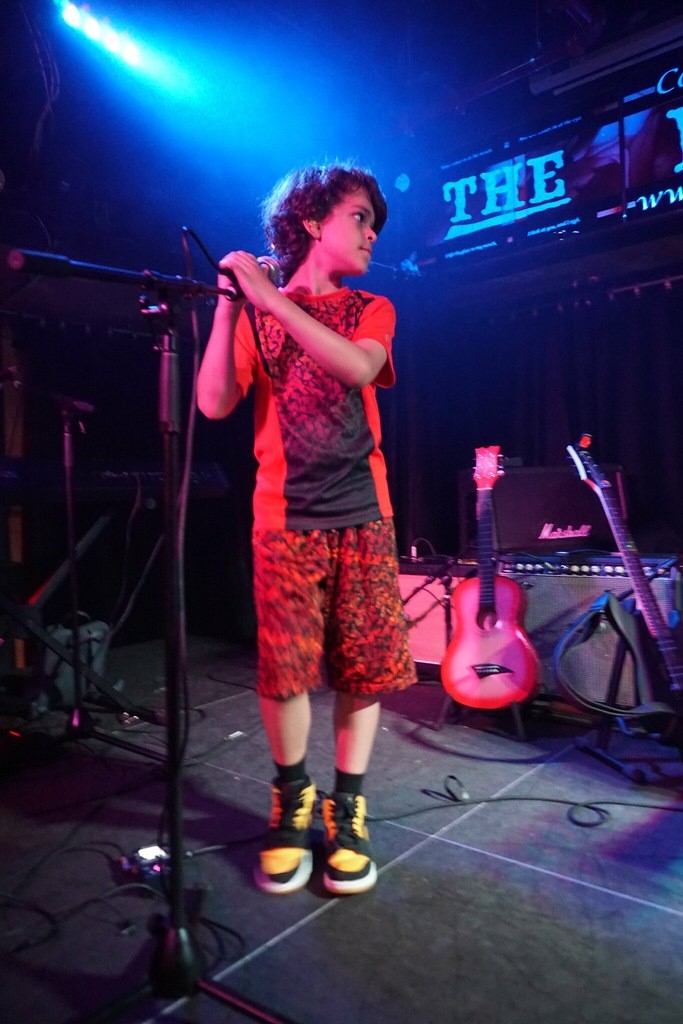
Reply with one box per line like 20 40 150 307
223 257 280 300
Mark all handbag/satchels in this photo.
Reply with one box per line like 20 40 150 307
43 611 111 705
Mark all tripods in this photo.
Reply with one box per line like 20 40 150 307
0 248 298 1024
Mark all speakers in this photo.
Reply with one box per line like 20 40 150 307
398 463 683 742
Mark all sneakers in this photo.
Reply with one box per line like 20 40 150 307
317 789 377 893
254 776 316 894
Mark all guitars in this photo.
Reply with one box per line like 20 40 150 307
564 432 683 692
438 446 540 711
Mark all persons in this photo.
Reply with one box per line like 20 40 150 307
198 162 418 894
